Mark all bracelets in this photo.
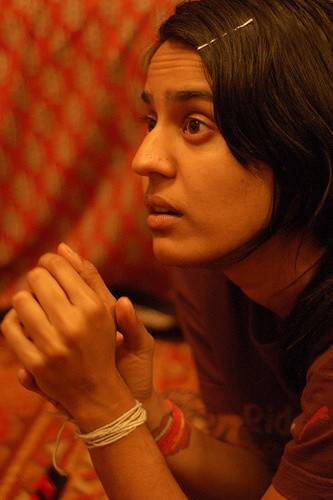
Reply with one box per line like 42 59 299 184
42 400 148 477
157 399 185 457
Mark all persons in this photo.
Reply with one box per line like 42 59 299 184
1 1 333 500
